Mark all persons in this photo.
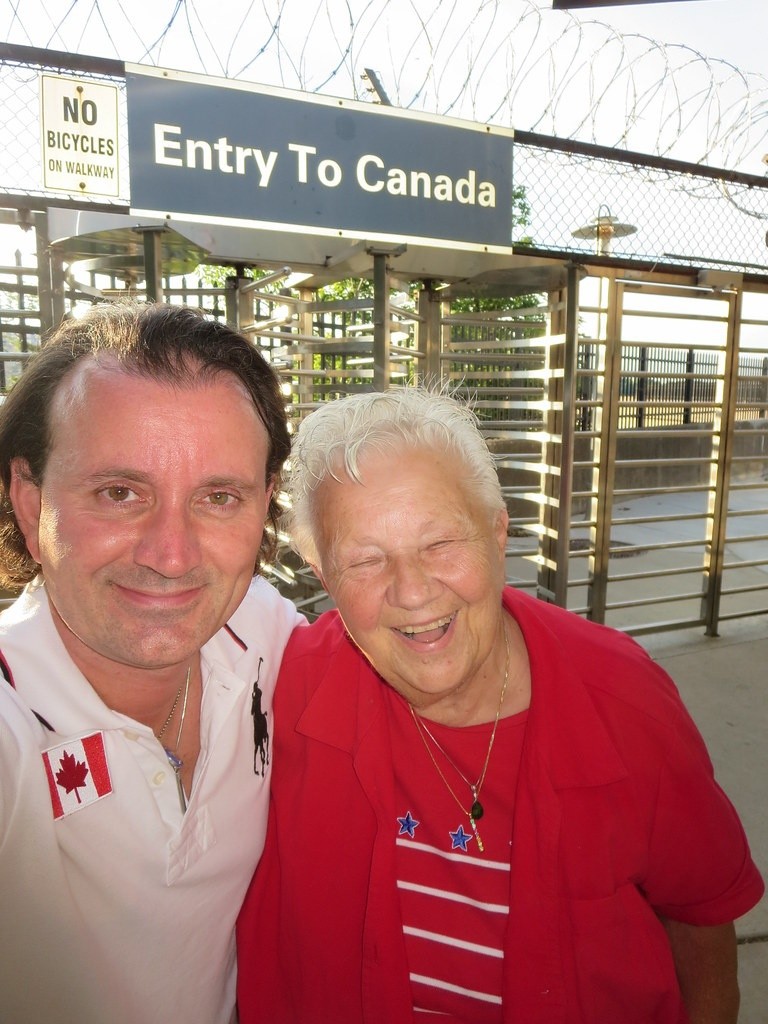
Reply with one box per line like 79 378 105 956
233 389 764 1024
0 305 312 1024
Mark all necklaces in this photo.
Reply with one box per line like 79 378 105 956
407 616 509 854
156 663 191 814
